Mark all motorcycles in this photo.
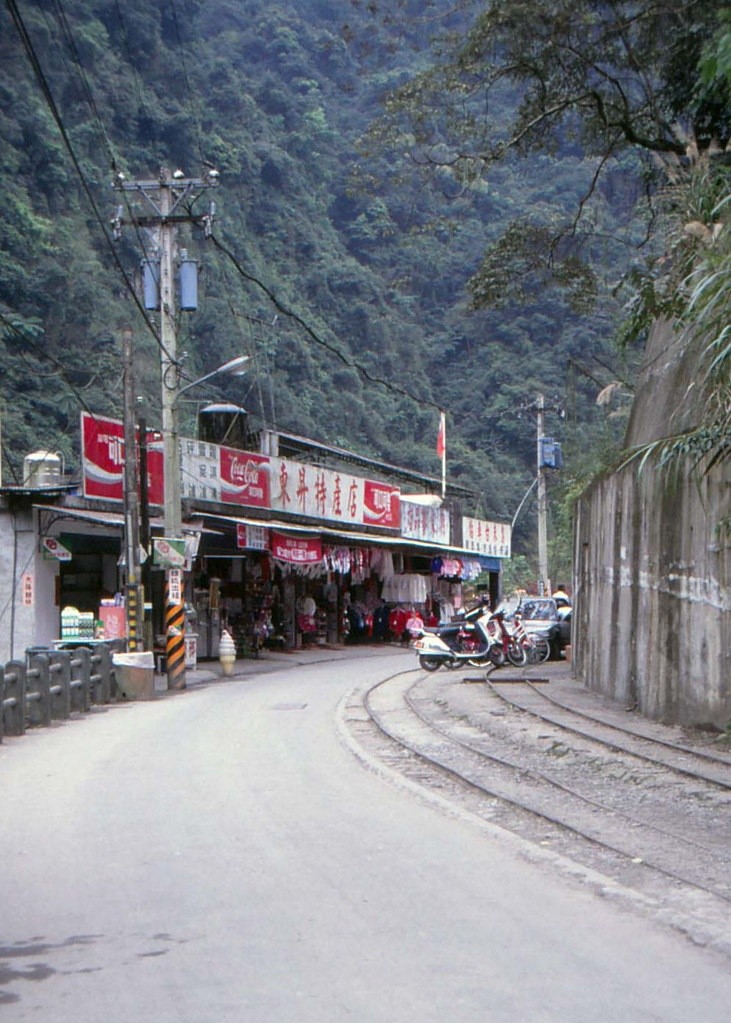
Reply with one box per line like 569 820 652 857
403 586 551 671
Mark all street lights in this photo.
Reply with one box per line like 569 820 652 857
152 355 255 696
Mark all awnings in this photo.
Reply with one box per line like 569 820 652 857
32 502 225 554
191 509 508 559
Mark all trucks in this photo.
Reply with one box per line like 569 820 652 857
493 596 570 661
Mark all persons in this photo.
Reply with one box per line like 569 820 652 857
552 584 569 604
